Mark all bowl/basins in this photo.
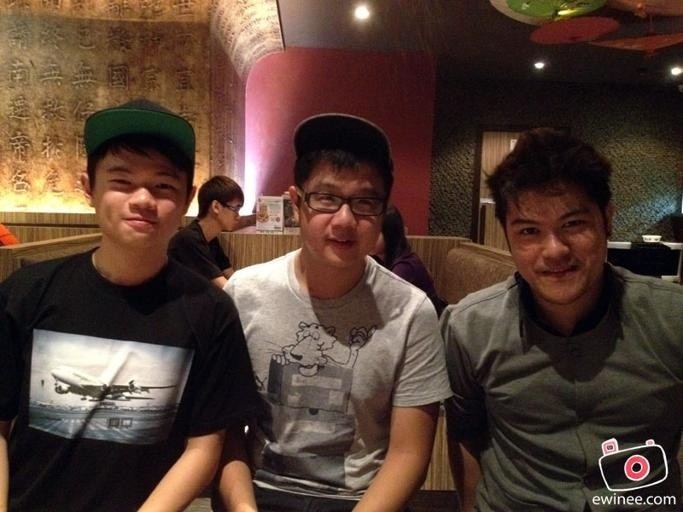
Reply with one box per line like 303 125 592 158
642 235 662 243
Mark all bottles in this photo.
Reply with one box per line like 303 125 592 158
282 192 292 227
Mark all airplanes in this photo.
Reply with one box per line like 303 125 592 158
48 367 177 402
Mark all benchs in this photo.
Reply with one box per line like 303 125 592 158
0 211 518 512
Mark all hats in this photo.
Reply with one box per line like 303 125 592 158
294 112 391 165
84 97 196 160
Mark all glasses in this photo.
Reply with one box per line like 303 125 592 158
222 203 240 215
295 182 386 217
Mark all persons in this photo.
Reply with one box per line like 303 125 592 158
438 129 683 511
170 174 245 290
0 98 259 511
211 111 455 512
369 202 436 302
